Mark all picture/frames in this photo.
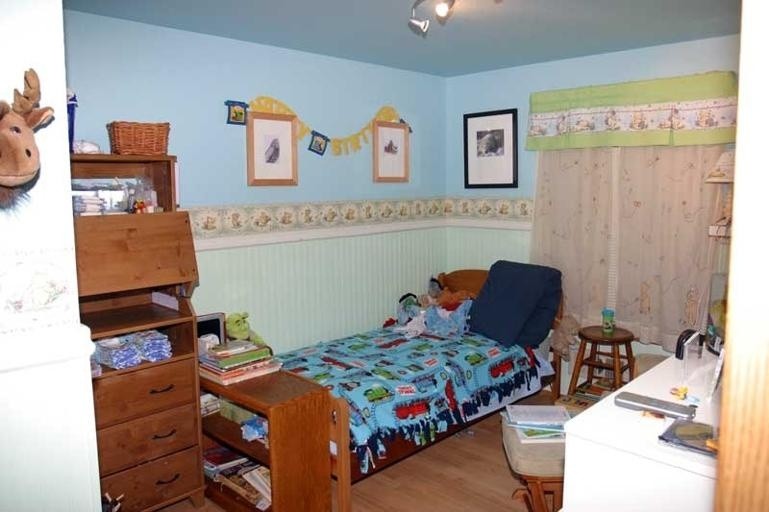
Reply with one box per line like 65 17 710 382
372 118 409 181
463 108 518 188
246 110 299 186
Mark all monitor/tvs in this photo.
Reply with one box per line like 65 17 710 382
196 312 227 346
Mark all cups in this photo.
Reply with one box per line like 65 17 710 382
601 310 614 336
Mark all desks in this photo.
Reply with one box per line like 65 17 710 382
566 325 634 396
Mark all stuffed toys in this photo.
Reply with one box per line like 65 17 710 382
395 274 481 342
0 68 55 215
222 310 265 353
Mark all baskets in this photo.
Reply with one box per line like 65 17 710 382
107 120 171 156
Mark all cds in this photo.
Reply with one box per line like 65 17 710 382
674 424 718 454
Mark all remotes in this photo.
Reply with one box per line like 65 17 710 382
614 391 696 420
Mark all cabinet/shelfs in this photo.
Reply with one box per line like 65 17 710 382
190 361 332 512
71 153 208 512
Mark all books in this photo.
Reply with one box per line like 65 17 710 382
197 334 280 386
501 401 579 445
575 378 629 402
199 441 273 511
197 393 225 417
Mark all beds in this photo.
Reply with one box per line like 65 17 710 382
266 269 564 512
